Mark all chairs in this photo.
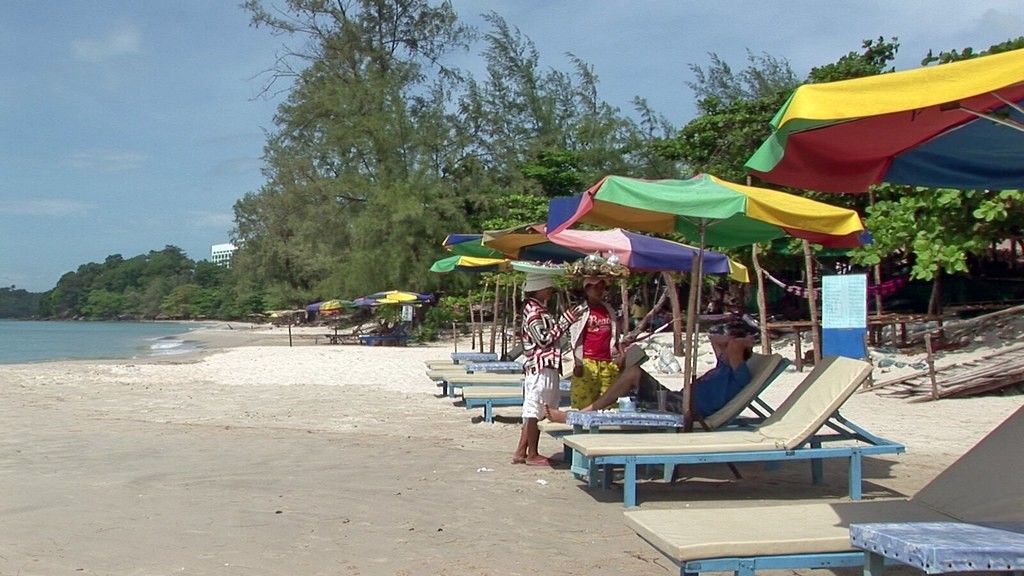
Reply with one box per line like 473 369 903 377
324 322 411 346
422 345 1023 575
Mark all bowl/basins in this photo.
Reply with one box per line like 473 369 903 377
619 402 635 412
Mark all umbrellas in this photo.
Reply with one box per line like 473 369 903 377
429 48 1024 419
352 290 434 344
272 302 354 325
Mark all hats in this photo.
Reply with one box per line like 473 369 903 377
521 273 555 292
583 278 609 288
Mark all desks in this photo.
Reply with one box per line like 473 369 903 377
466 361 522 374
846 522 1023 576
563 410 682 488
450 352 499 364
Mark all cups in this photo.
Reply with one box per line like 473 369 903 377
656 390 667 411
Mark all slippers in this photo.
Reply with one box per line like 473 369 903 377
526 457 556 465
511 454 528 464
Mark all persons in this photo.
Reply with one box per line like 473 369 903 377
543 323 761 424
630 299 648 322
295 314 301 326
704 296 721 315
568 273 626 411
304 311 308 323
510 274 583 466
